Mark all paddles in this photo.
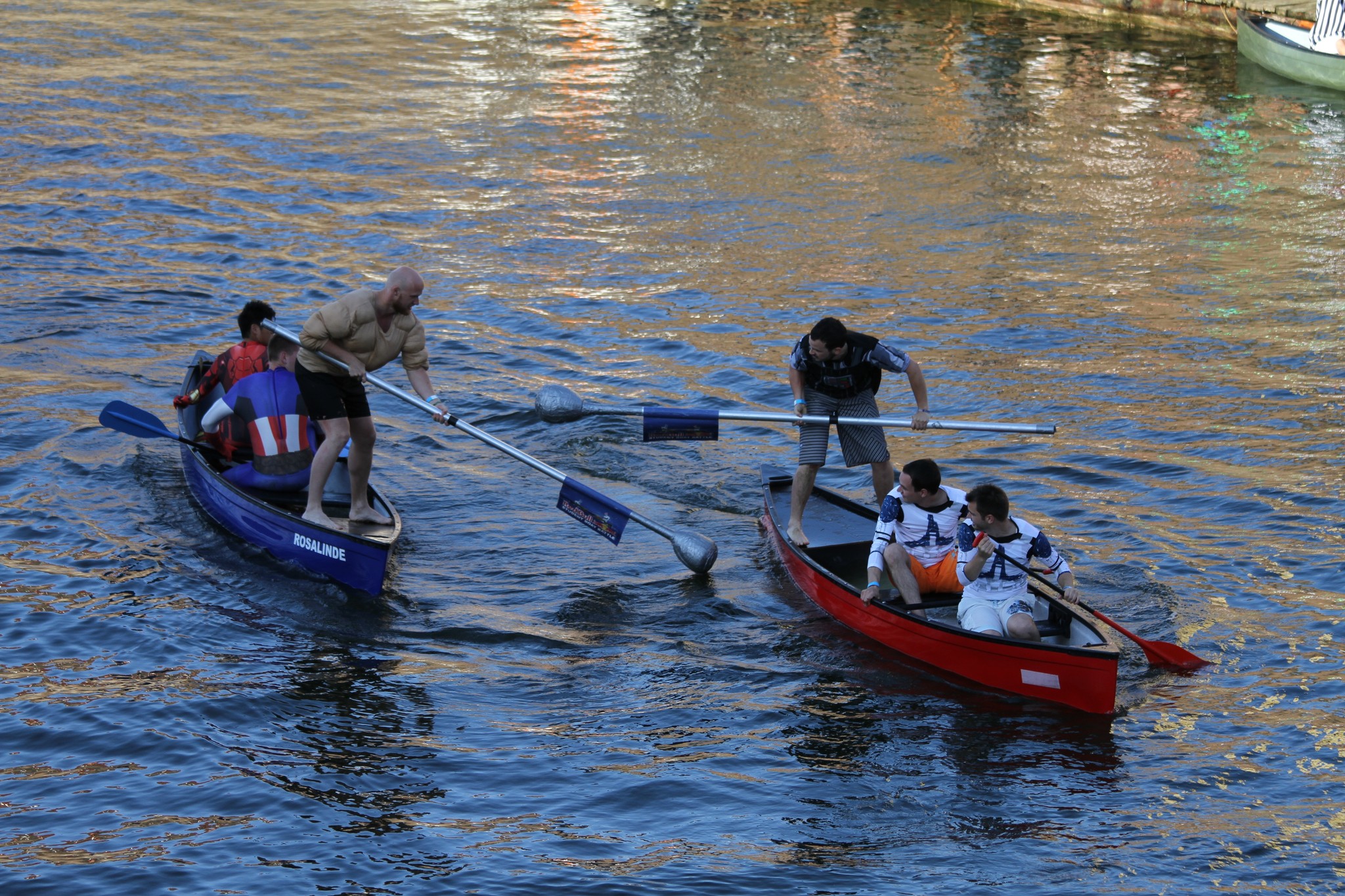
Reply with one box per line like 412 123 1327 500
94 395 222 459
968 532 1212 673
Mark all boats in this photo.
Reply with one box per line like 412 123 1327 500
178 349 401 597
757 462 1122 724
1235 6 1345 93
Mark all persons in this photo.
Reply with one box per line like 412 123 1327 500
955 484 1081 643
173 300 276 462
786 317 930 546
860 458 970 620
201 333 316 492
295 266 450 530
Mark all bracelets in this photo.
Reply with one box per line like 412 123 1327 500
866 581 880 588
425 395 442 406
1062 586 1074 589
794 399 807 406
918 409 930 414
